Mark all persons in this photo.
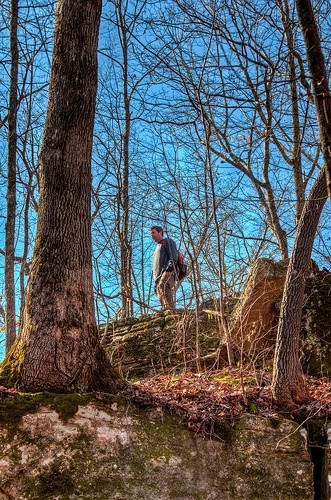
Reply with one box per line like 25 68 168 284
150 226 180 311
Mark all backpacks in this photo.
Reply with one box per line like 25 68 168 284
161 238 186 281
